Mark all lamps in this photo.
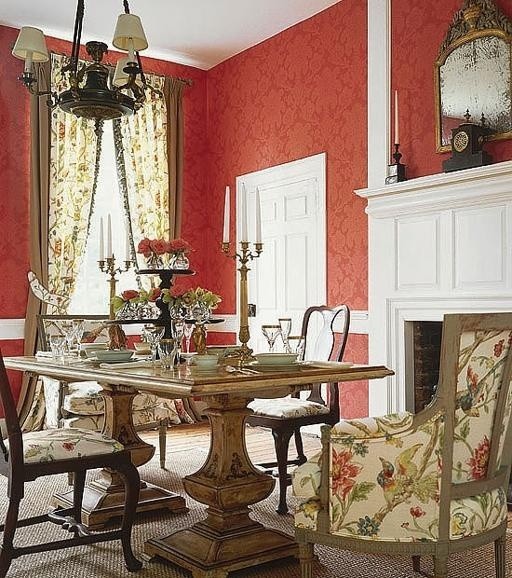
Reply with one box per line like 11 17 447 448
12 0 162 135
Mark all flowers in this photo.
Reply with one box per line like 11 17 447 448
112 286 221 320
168 239 195 254
138 237 167 255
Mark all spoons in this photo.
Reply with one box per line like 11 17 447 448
226 365 252 375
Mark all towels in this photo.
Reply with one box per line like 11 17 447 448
99 359 161 370
296 360 354 369
35 350 78 359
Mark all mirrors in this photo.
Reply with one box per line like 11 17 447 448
432 0 512 154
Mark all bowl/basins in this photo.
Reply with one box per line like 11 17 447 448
133 342 151 350
95 351 134 360
256 352 299 365
194 358 218 366
84 348 107 358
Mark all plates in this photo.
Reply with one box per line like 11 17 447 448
253 363 299 371
91 359 128 364
180 353 197 357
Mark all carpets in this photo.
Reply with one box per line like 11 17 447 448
0 412 510 577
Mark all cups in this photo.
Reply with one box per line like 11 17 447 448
158 339 177 368
287 336 301 364
51 334 66 358
62 326 75 358
261 325 282 352
279 318 291 353
297 336 305 362
50 328 59 362
171 320 185 367
184 324 193 353
145 327 165 365
73 320 84 358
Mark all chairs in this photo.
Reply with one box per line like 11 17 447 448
244 305 352 516
38 313 177 486
293 314 512 578
0 351 143 578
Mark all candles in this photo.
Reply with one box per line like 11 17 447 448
394 90 399 146
99 217 104 260
125 220 130 260
241 184 248 241
223 186 229 241
255 188 262 243
107 214 112 257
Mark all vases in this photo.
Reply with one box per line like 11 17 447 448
148 254 163 269
169 252 189 270
